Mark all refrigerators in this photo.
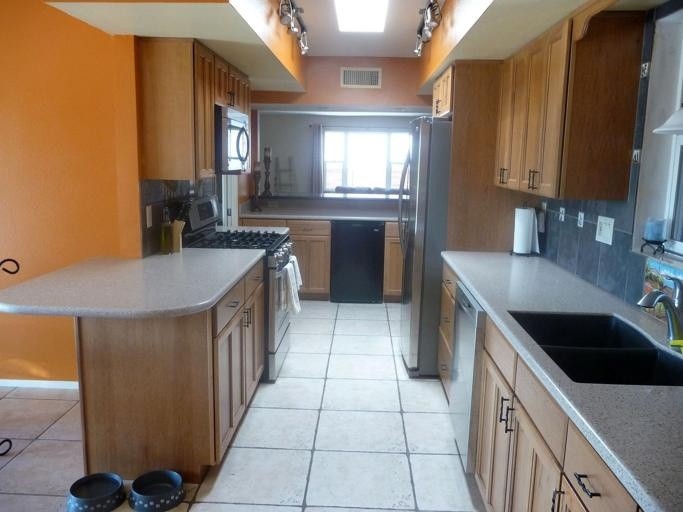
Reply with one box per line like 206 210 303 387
399 116 451 379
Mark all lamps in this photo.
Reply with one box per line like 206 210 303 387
276 1 310 58
412 1 443 58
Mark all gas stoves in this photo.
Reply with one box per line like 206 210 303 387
180 230 290 249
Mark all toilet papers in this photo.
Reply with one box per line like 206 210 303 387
513 206 540 255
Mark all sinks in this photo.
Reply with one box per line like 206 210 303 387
538 346 683 387
506 310 657 349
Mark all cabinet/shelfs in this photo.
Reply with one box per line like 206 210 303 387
433 63 516 118
241 218 287 227
518 6 646 201
288 219 330 302
214 54 253 115
550 418 644 512
474 309 570 512
434 260 459 406
138 37 216 178
490 46 527 192
74 259 269 482
384 221 407 301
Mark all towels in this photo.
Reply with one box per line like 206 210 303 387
280 263 301 313
289 255 303 290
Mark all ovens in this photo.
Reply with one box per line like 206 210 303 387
264 241 293 383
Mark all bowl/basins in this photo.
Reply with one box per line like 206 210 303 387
64 471 126 512
127 469 186 511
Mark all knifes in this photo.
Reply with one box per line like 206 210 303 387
175 201 192 221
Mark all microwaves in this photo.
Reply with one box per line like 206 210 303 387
216 105 249 173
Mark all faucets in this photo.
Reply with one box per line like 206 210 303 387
635 275 683 349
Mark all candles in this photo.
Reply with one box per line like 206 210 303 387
263 143 271 161
254 162 261 172
